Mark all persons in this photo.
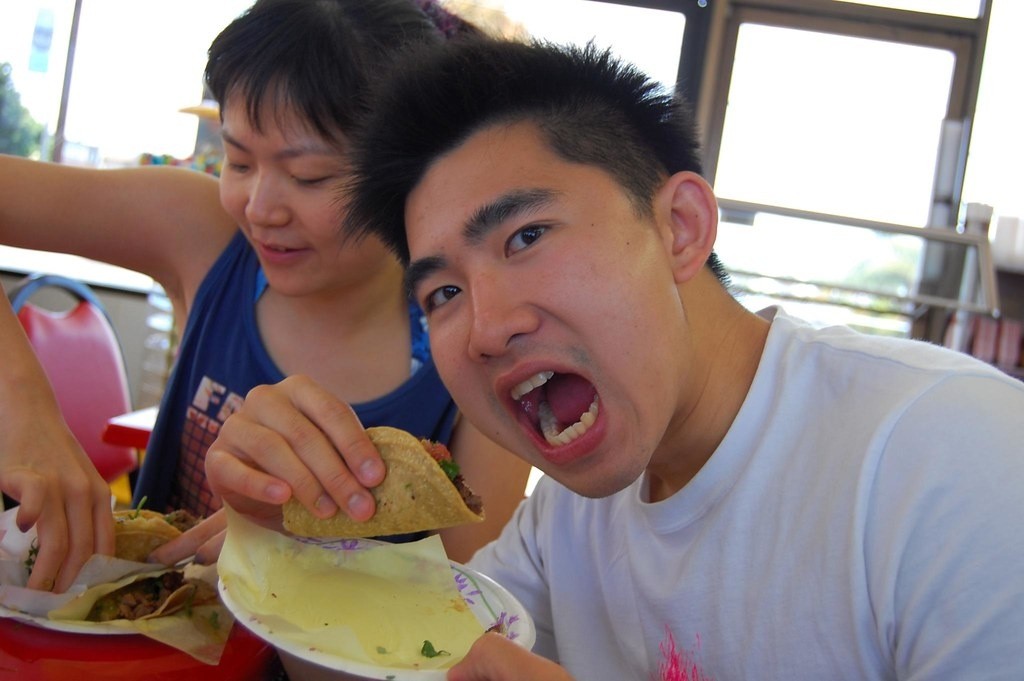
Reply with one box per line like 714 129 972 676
205 41 1024 681
0 0 531 592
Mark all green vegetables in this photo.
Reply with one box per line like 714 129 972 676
23 496 147 575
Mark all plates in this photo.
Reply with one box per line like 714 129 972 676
218 535 536 680
0 598 227 636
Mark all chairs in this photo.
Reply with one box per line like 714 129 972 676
9 274 141 510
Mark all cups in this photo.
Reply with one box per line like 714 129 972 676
966 204 994 237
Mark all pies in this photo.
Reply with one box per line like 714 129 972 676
106 509 183 568
281 427 486 537
46 569 218 620
